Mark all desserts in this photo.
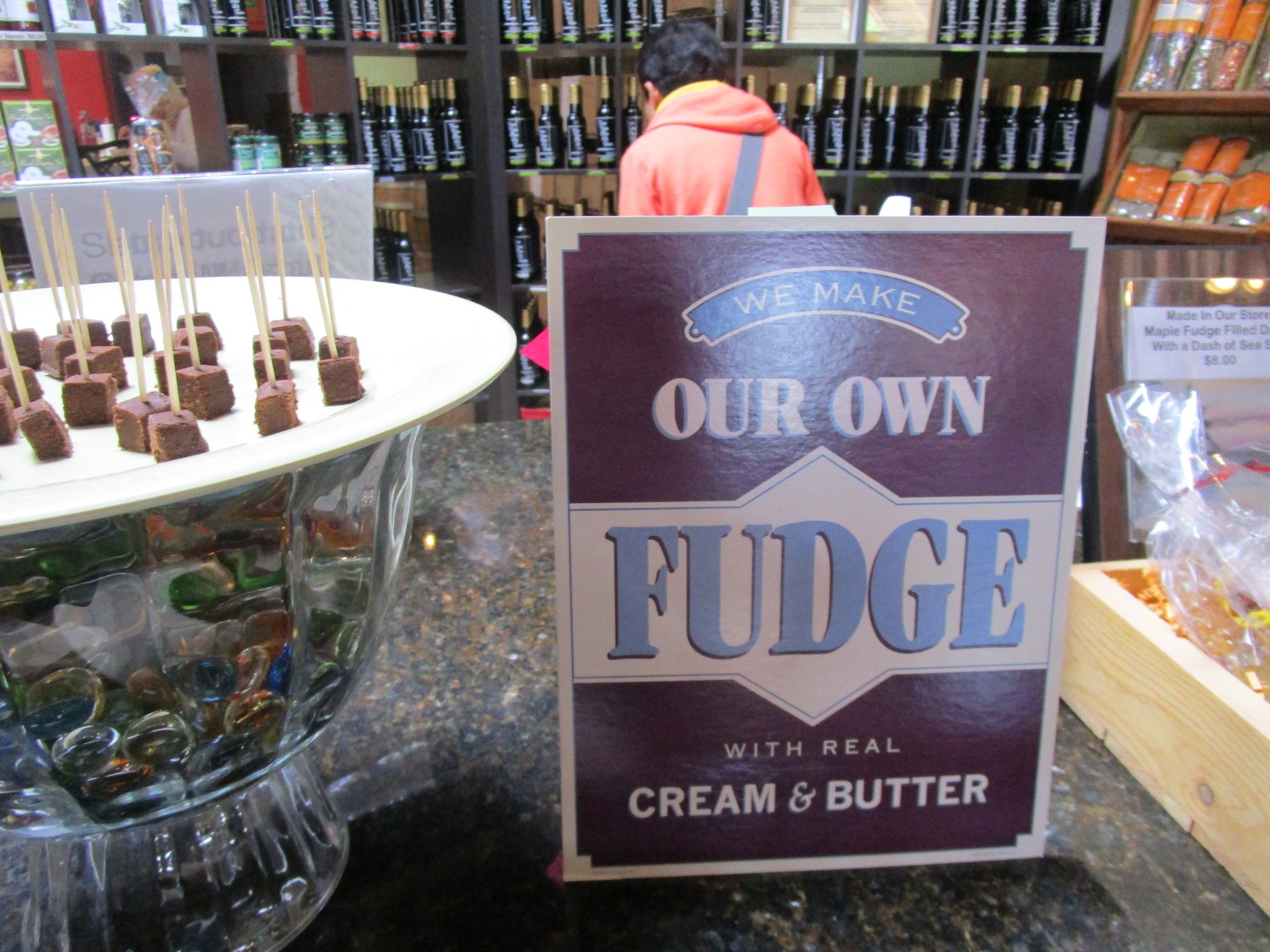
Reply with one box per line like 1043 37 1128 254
0 311 365 461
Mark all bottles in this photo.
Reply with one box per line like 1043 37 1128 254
511 192 616 283
209 1 785 44
827 198 1062 217
372 207 417 287
934 0 1110 46
357 77 1084 171
518 295 547 387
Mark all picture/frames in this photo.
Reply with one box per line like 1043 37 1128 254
0 44 28 90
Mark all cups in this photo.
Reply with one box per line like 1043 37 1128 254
0 423 422 952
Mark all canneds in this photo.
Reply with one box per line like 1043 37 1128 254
289 111 352 165
228 135 283 171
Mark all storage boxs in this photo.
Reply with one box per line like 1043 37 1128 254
0 99 70 197
1058 557 1270 915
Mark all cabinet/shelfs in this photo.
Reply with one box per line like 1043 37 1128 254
1091 1 1270 247
0 0 482 302
482 0 1129 423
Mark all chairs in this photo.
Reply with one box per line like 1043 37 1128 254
78 137 131 179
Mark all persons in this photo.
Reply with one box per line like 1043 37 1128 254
618 21 827 216
149 79 202 175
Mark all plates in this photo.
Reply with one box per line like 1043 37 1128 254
0 275 517 533
